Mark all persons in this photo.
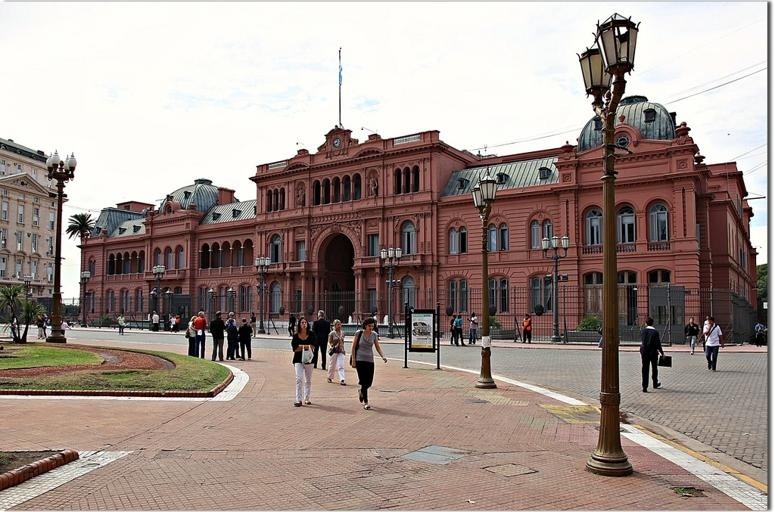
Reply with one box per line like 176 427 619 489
288 313 296 337
36 313 72 340
295 184 305 205
312 309 331 371
147 310 181 333
683 314 724 372
450 315 456 344
467 311 478 344
327 319 348 386
453 314 467 347
351 317 387 410
754 322 765 347
290 317 317 406
117 313 125 335
639 317 665 393
522 313 532 344
370 173 378 196
188 309 257 361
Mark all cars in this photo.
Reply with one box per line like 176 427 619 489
44 312 70 327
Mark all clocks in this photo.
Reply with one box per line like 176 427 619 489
333 138 341 147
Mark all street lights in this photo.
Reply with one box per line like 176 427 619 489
45 150 77 342
541 234 570 342
152 264 166 330
80 270 90 327
575 13 642 478
379 244 404 338
254 254 272 334
23 273 33 325
469 165 501 388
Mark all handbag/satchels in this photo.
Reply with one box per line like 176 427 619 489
350 355 355 365
185 328 190 338
